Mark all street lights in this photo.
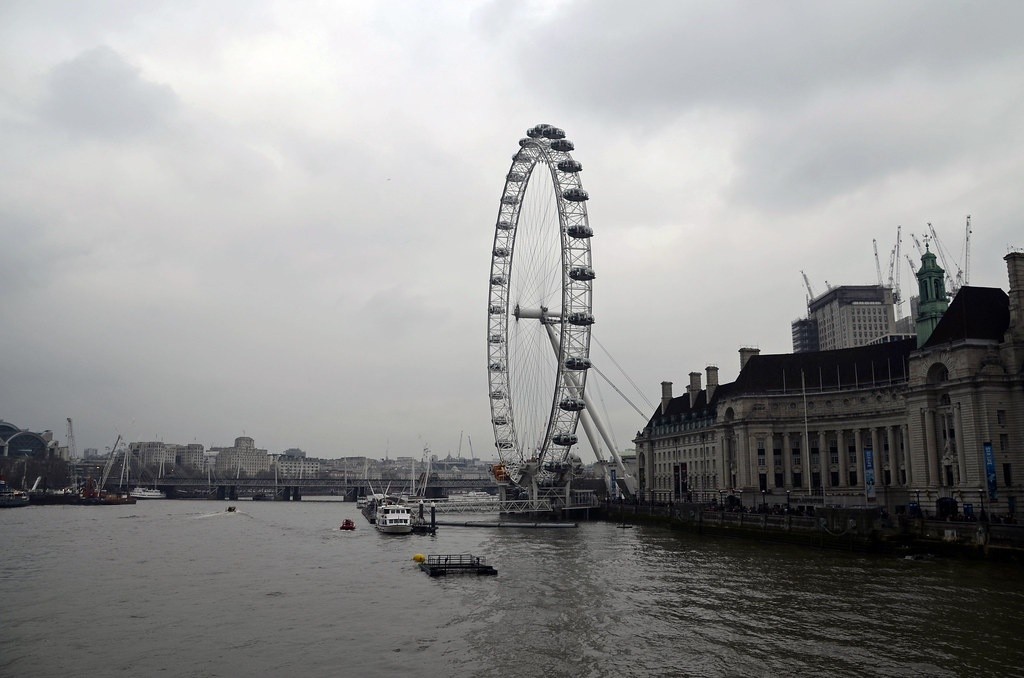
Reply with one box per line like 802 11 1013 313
651 489 654 506
718 489 723 507
977 485 985 513
914 487 921 519
786 488 790 513
668 489 672 506
761 489 767 513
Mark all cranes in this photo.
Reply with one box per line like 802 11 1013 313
796 213 973 334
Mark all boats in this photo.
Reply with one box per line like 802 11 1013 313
417 551 498 578
225 505 237 514
339 517 356 531
120 478 167 500
367 479 416 534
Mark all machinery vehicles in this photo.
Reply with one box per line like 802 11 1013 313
64 417 123 501
